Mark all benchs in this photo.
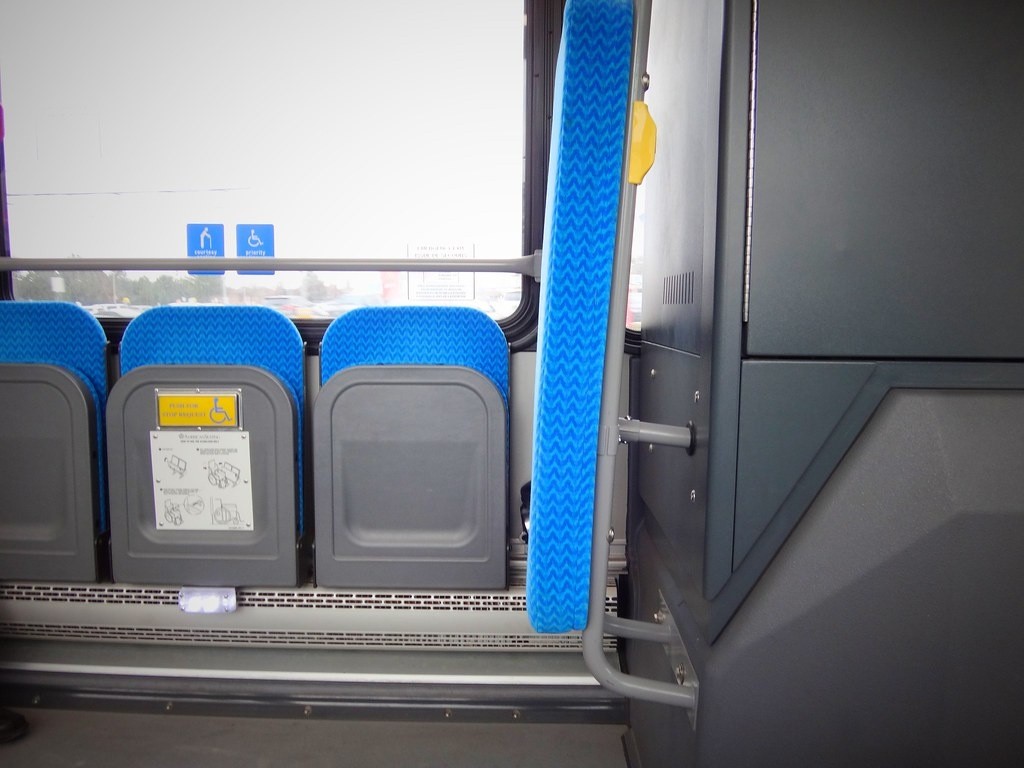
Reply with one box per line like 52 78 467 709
0 303 509 593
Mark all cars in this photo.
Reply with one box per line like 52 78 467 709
265 294 328 317
502 289 523 311
